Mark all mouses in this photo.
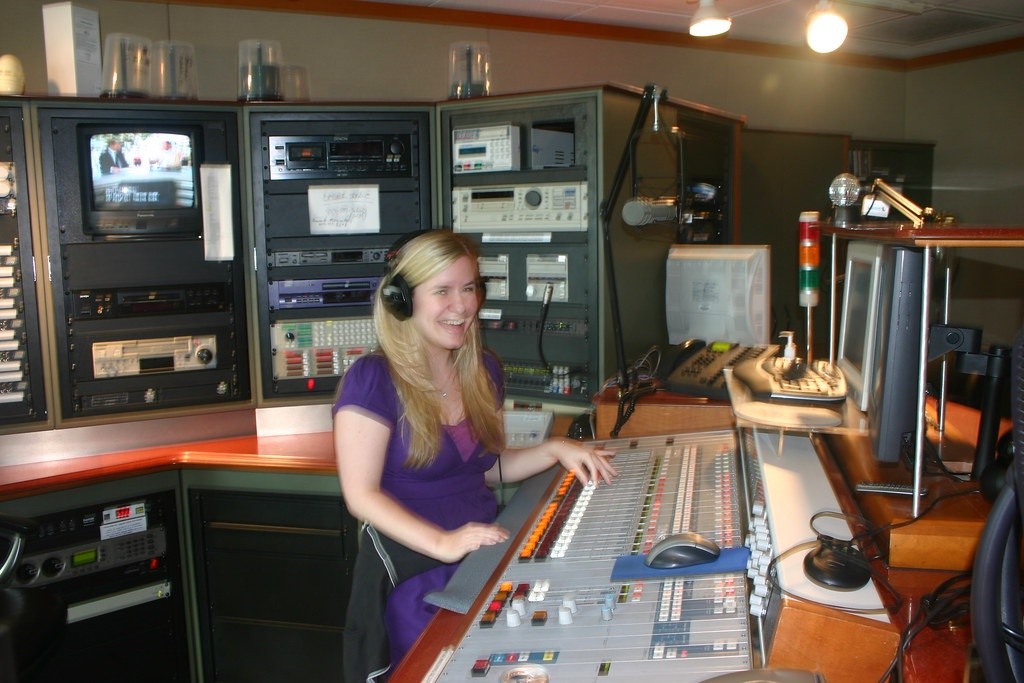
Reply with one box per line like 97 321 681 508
645 532 721 569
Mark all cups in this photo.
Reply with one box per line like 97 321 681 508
280 64 310 102
238 40 284 103
447 41 491 99
101 33 152 98
153 42 198 101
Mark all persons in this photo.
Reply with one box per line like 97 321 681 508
332 227 618 683
160 140 182 168
98 138 129 174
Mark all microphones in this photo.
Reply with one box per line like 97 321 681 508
622 198 677 226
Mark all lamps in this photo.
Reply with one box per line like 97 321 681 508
688 0 732 38
804 0 848 54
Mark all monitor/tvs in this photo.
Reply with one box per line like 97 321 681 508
836 238 1011 482
77 123 205 242
665 245 771 346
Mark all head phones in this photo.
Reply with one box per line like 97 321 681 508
380 229 486 321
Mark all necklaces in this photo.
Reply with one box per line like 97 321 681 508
441 392 448 399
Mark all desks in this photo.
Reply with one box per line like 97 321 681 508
0 387 995 683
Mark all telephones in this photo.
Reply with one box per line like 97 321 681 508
658 341 782 398
617 357 656 397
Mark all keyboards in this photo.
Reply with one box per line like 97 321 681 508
732 355 848 402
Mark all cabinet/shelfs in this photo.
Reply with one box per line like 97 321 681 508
818 220 1024 573
